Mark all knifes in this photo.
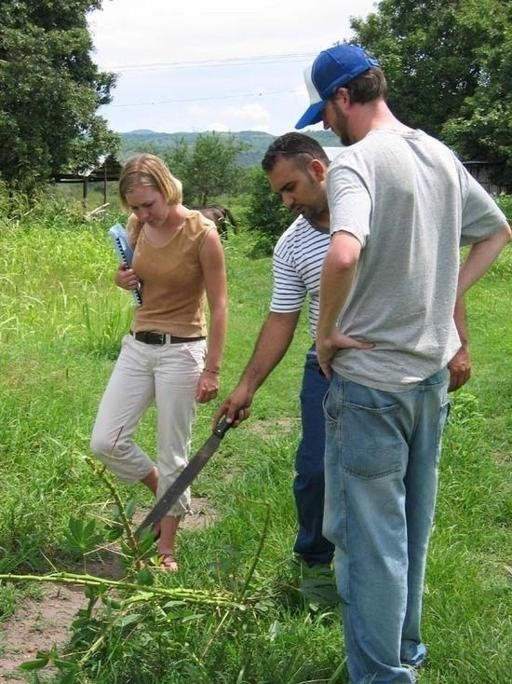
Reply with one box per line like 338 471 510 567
128 408 245 549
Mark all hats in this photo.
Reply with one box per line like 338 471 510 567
295 43 380 128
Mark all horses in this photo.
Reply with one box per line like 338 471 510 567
183 201 239 245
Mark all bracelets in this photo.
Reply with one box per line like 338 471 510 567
202 366 220 375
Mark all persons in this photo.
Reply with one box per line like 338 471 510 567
89 153 229 575
291 43 512 684
211 130 472 568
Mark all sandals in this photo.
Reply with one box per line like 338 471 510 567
158 553 178 572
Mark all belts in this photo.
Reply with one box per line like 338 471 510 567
130 328 206 344
